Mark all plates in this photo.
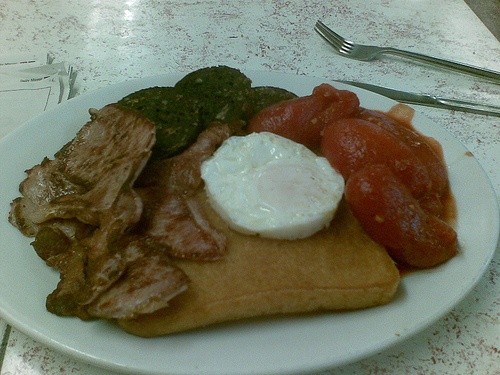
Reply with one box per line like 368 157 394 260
1 70 499 375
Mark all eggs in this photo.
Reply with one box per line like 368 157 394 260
202 131 345 239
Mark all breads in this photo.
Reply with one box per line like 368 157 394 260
114 189 400 337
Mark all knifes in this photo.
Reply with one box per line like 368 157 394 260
332 80 500 117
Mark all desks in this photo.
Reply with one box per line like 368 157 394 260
0 0 500 375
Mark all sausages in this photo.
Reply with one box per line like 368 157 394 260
344 164 459 268
250 90 447 207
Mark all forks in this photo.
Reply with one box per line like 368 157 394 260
314 19 500 85
